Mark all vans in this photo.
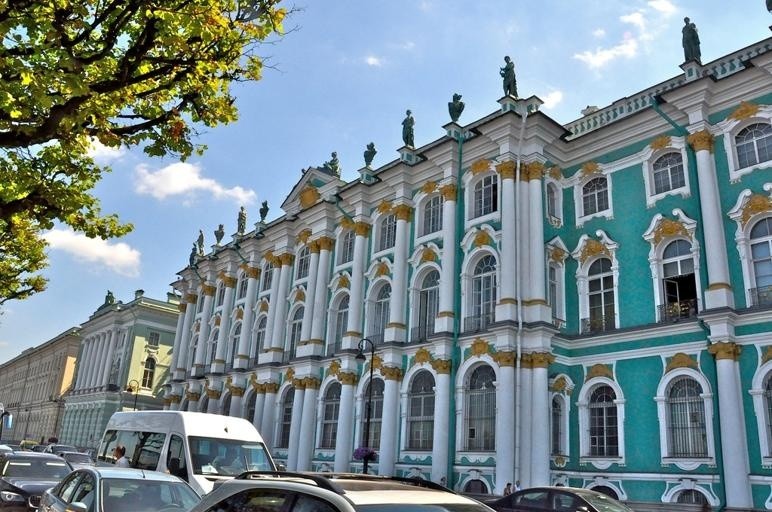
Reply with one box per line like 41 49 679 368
95 410 280 499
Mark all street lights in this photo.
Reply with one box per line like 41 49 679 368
127 379 140 411
356 338 374 475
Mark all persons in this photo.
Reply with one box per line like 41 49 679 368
238 205 247 237
189 241 197 266
503 482 512 497
197 229 206 258
440 476 446 486
214 447 245 474
112 444 131 468
401 110 415 147
513 480 522 493
323 151 340 174
681 16 701 61
498 55 520 100
